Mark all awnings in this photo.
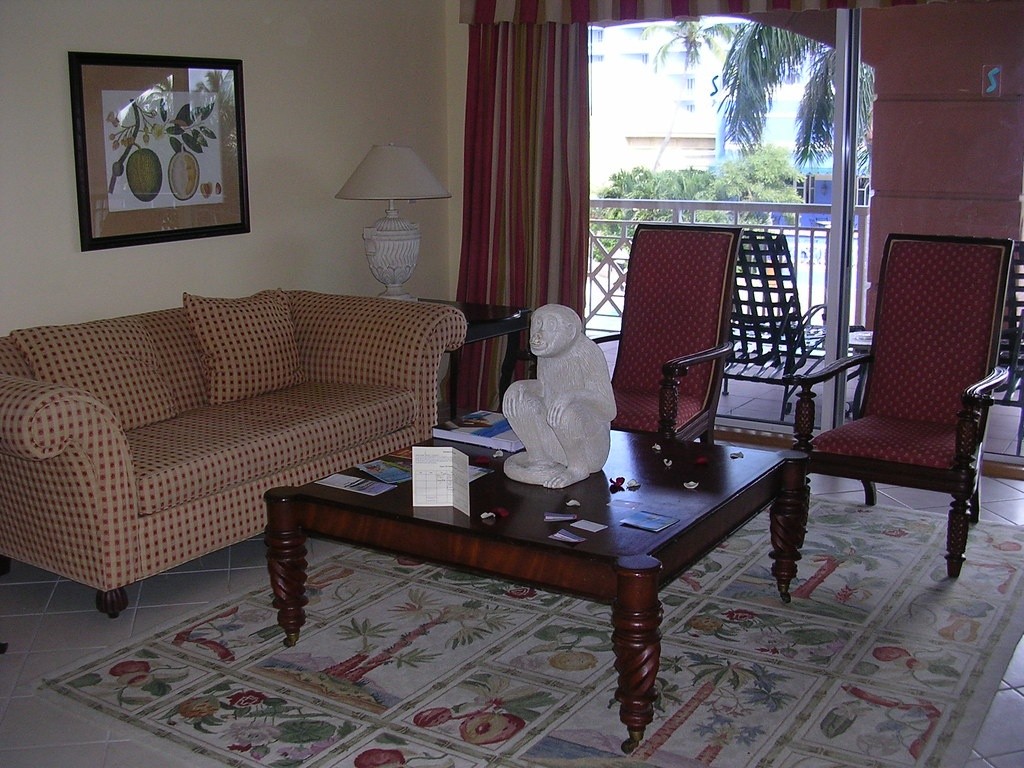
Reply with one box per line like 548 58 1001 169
708 150 833 175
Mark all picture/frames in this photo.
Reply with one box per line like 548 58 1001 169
68 51 251 252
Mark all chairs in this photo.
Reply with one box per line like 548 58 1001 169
723 231 867 432
995 240 1024 458
591 223 745 444
782 233 1015 578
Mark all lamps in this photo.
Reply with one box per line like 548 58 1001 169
335 143 452 301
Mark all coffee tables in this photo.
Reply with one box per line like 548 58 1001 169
262 429 811 754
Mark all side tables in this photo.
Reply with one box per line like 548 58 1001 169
417 298 530 421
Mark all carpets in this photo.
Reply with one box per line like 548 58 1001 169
29 494 1023 768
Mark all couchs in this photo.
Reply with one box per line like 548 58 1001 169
0 287 469 619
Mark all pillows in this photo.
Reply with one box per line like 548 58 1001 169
9 316 183 433
183 287 306 406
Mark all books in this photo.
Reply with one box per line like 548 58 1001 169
432 410 525 453
